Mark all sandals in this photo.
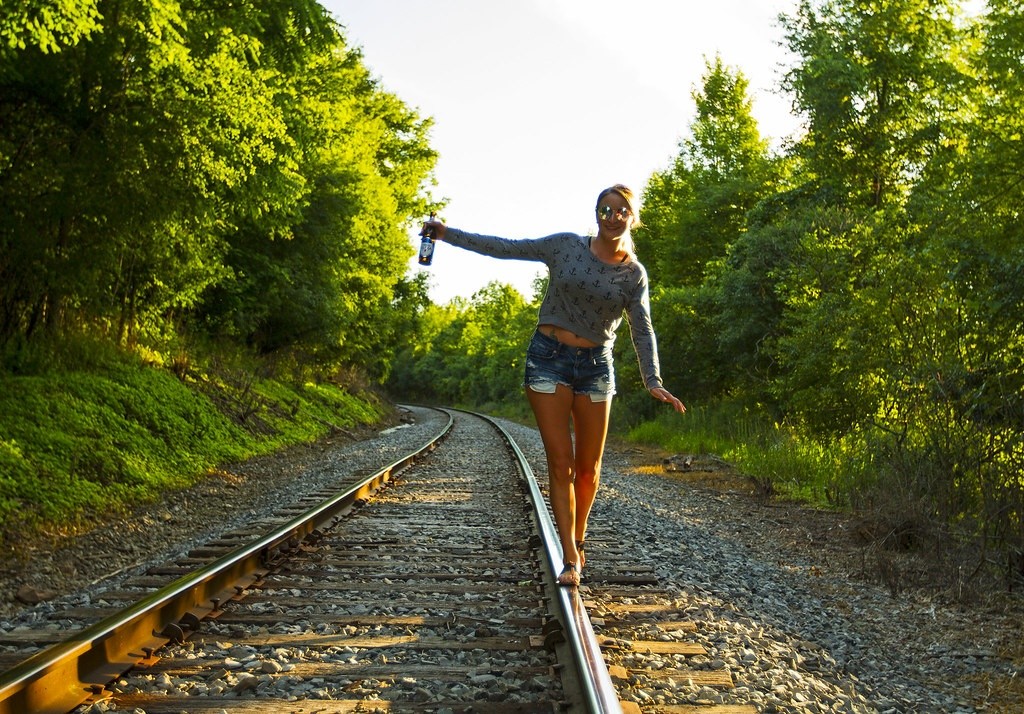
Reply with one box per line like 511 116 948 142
573 540 586 569
555 559 581 587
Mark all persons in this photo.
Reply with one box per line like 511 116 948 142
417 183 687 586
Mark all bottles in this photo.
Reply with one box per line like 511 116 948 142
419 211 437 265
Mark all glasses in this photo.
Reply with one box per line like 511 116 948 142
597 205 632 222
